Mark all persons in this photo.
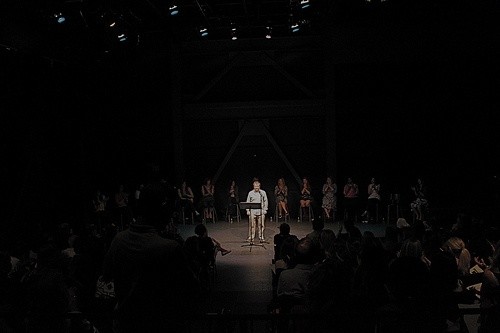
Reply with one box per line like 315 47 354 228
177 179 200 221
322 176 336 223
273 213 500 333
226 180 239 222
409 178 433 223
343 177 359 220
0 220 224 333
367 177 381 220
296 177 318 223
201 176 215 220
275 177 289 219
88 180 148 237
245 178 269 243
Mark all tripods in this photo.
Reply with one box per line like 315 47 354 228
239 191 270 252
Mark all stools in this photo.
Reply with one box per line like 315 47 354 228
412 207 422 225
344 205 359 225
387 193 401 225
179 206 194 225
275 203 290 223
323 208 335 224
367 203 380 224
93 211 130 230
228 203 241 224
201 207 217 224
301 199 312 224
